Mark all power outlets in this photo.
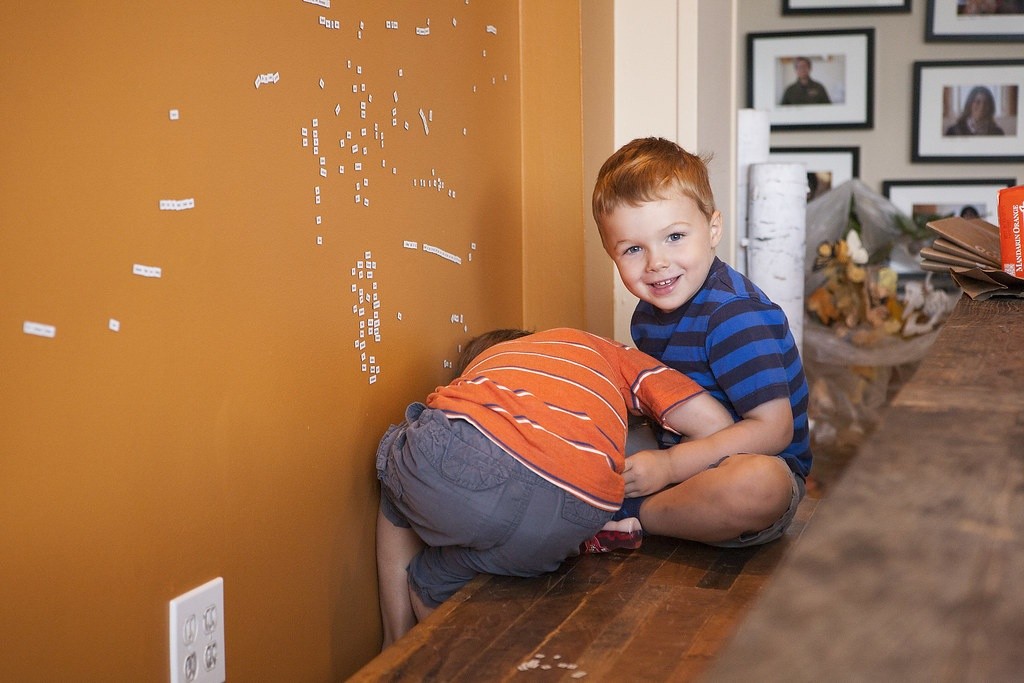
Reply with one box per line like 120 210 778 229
168 577 226 683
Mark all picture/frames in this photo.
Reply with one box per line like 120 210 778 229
882 178 1017 280
923 0 1024 44
745 27 875 130
768 146 860 204
781 0 911 17
911 58 1024 164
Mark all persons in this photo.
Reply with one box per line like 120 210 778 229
591 138 814 547
782 57 831 105
376 327 734 654
944 85 1006 137
